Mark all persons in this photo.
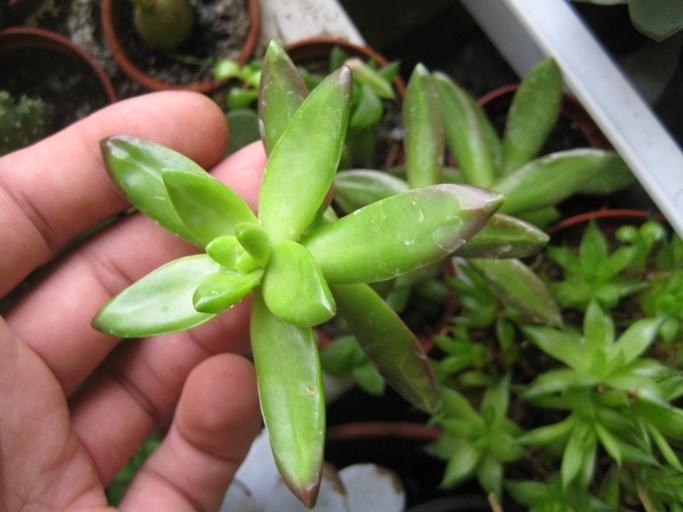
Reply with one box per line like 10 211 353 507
1 89 335 511
